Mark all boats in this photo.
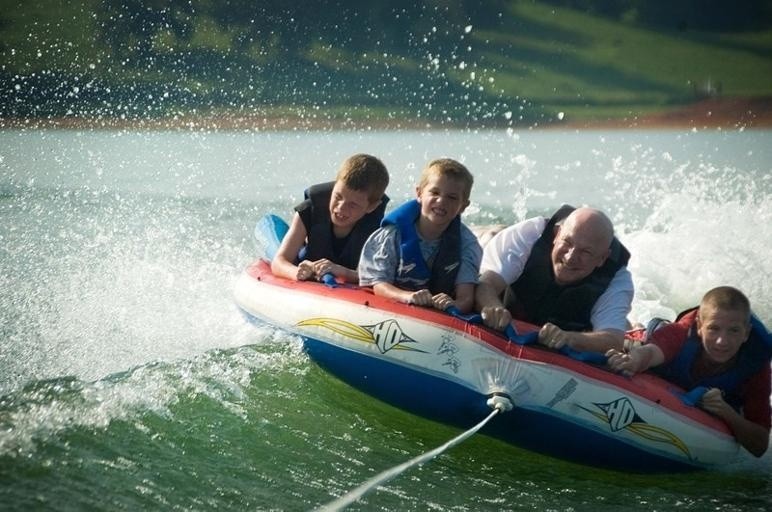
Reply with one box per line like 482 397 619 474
235 259 738 478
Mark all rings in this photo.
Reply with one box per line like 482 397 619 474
551 339 557 344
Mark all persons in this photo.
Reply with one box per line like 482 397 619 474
270 154 396 285
605 286 772 459
358 158 483 316
474 204 634 357
